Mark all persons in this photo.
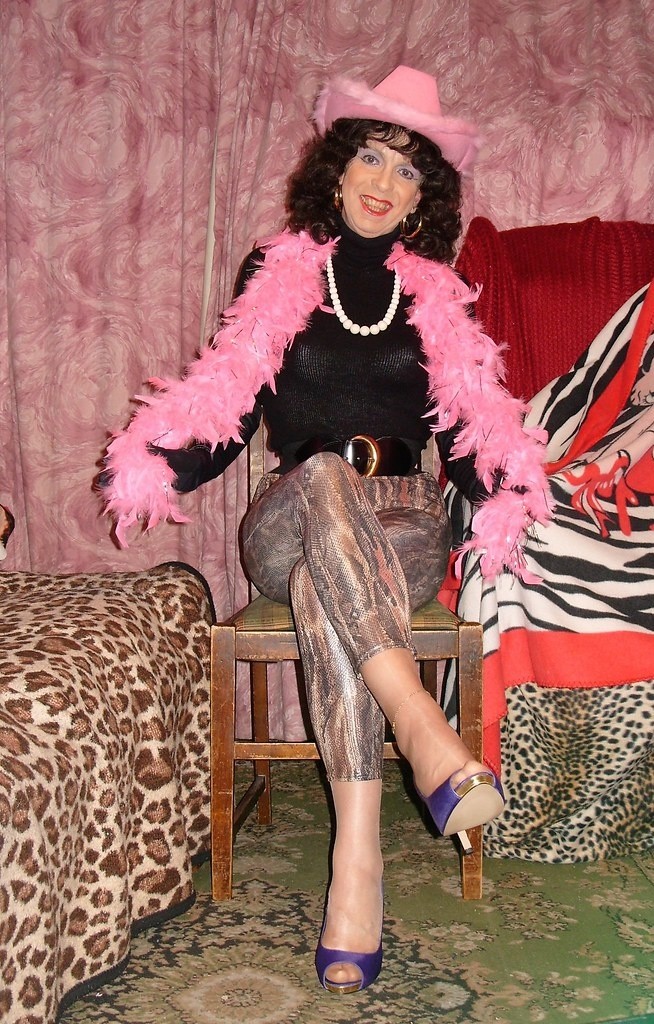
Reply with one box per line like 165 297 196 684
101 66 556 991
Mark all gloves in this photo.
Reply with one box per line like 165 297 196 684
471 468 528 509
95 442 202 493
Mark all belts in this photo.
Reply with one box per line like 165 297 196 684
279 435 412 477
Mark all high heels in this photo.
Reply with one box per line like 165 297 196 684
413 760 505 855
314 876 384 993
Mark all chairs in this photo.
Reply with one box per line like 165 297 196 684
438 215 654 857
208 414 485 902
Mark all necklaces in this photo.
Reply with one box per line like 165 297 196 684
325 254 399 334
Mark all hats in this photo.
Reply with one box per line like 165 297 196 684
308 64 477 171
